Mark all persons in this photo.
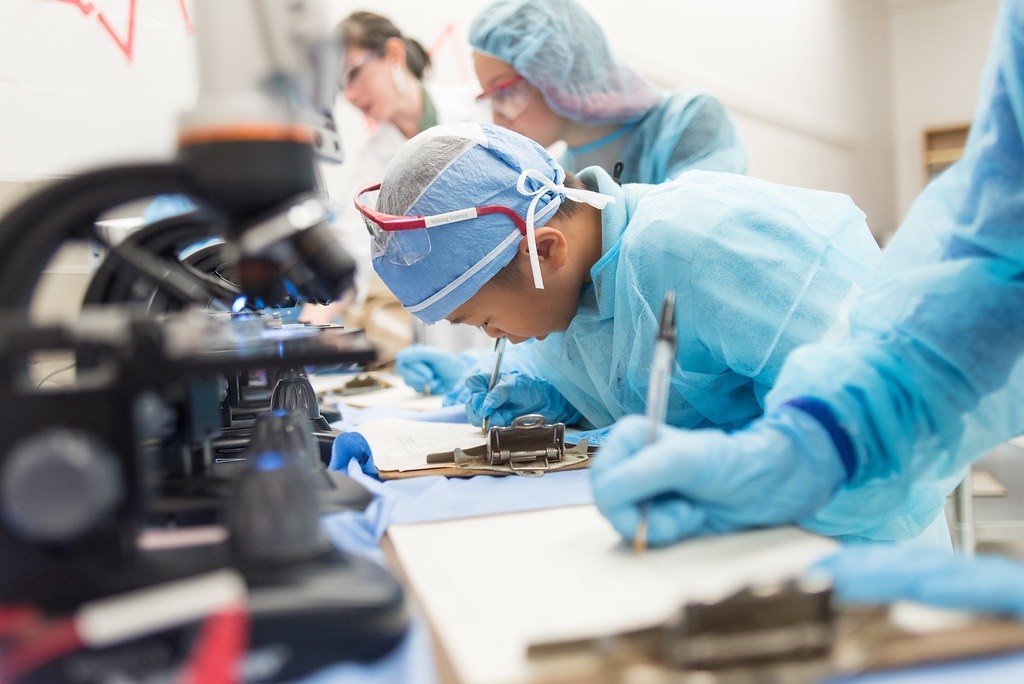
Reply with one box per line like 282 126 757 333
137 0 745 411
588 0 1024 623
369 122 967 555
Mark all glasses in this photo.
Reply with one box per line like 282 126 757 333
352 182 529 267
474 75 528 121
335 48 383 92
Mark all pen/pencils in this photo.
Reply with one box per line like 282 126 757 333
629 290 679 559
482 336 507 438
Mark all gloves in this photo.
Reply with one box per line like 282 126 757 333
394 343 477 396
587 405 847 546
464 371 583 433
